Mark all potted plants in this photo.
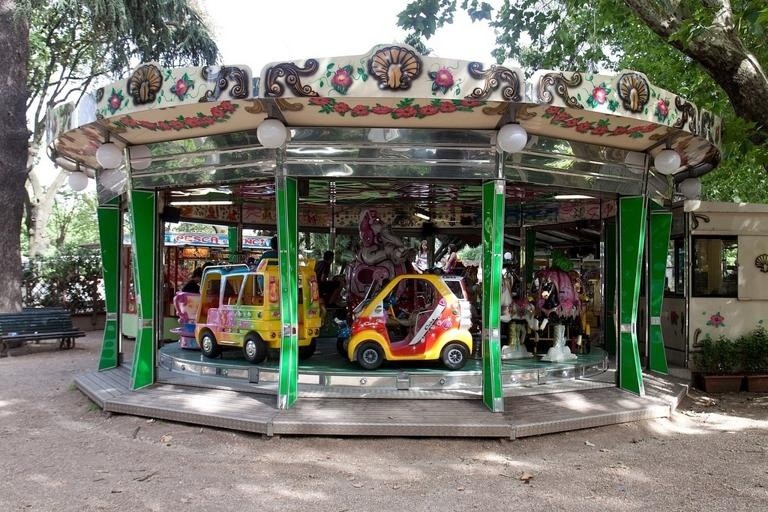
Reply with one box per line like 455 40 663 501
698 327 768 394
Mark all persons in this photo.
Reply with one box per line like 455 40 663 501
372 278 397 318
181 262 234 300
412 240 456 275
312 252 348 308
255 238 281 296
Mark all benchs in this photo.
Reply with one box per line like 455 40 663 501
0 308 86 356
